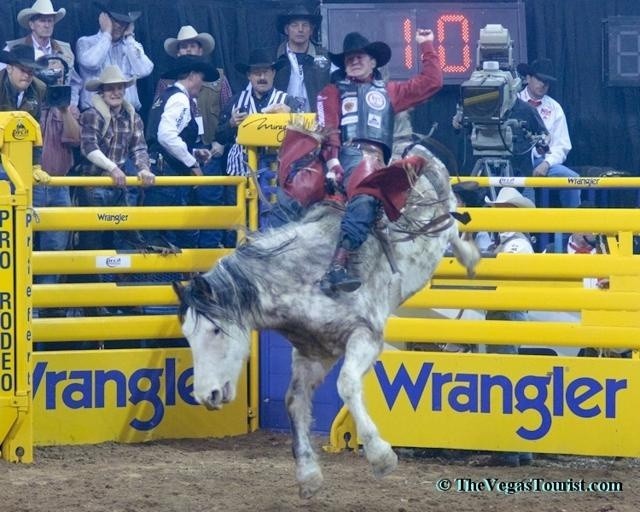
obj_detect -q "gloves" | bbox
[325,158,345,183]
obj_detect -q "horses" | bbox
[170,139,481,501]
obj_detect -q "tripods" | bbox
[469,119,553,244]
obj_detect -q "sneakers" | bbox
[321,268,362,295]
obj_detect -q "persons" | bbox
[0,1,639,358]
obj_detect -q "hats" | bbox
[485,186,535,207]
[86,64,135,91]
[451,181,489,209]
[159,53,220,81]
[518,57,559,82]
[327,31,391,70]
[98,1,149,23]
[235,47,289,76]
[0,45,44,69]
[163,25,215,58]
[277,5,323,35]
[17,1,66,29]
